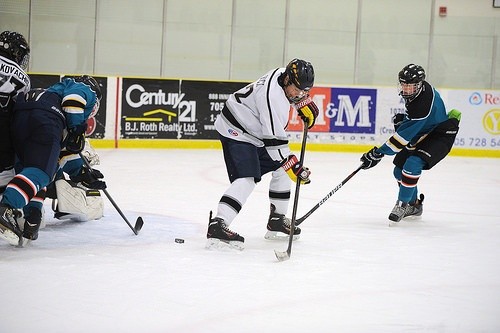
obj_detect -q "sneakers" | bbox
[206,210,245,252]
[388,201,407,227]
[265,204,301,240]
[0,203,23,246]
[19,209,43,247]
[403,194,424,218]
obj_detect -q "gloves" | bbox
[280,155,311,185]
[74,167,106,189]
[393,113,406,132]
[294,97,319,128]
[359,146,384,169]
[64,121,88,153]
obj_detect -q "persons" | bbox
[0,30,104,223]
[206,57,320,253]
[0,74,106,247]
[361,63,461,227]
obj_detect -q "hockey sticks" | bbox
[295,164,365,226]
[274,122,308,261]
[78,151,144,235]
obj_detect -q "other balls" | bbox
[175,239,184,243]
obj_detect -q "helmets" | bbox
[0,31,30,70]
[398,64,425,100]
[286,58,315,103]
[76,74,101,99]
